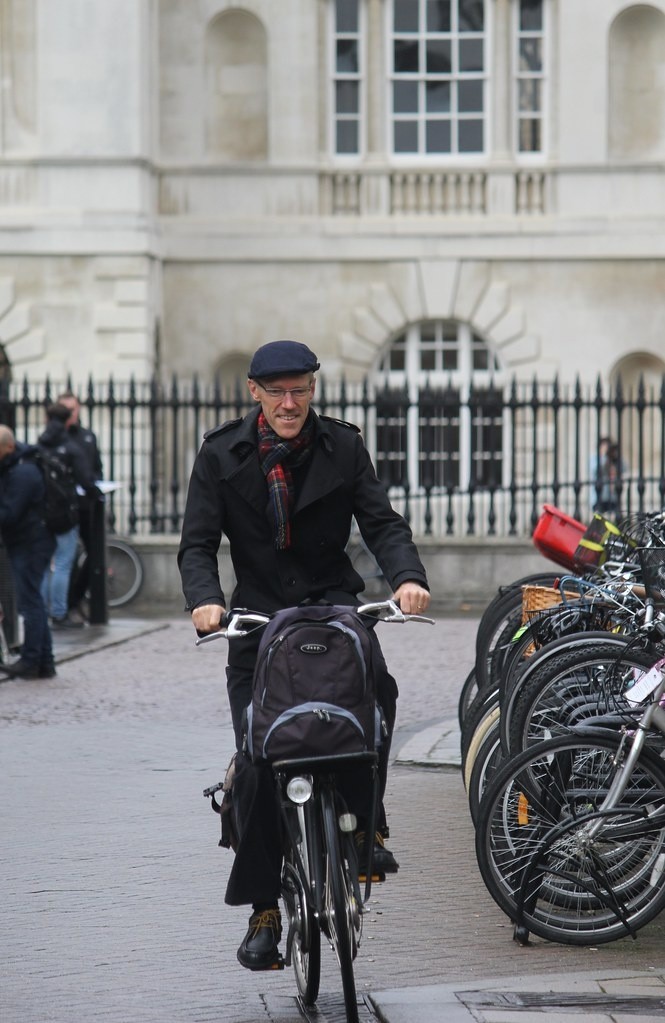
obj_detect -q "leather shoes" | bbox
[236,908,283,971]
[345,829,399,874]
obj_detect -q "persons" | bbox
[35,403,84,632]
[58,393,109,630]
[0,425,81,679]
[176,341,432,971]
[591,438,623,523]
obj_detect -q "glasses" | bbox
[255,380,312,395]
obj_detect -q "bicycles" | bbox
[78,539,142,609]
[194,597,437,1023]
[346,526,395,605]
[458,502,664,947]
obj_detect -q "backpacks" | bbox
[6,446,79,535]
[248,592,389,770]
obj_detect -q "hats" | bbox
[247,339,320,378]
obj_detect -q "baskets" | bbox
[635,545,665,602]
[603,530,647,572]
[525,601,615,652]
[569,512,639,570]
[518,583,611,662]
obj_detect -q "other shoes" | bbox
[1,653,56,680]
[49,614,86,632]
